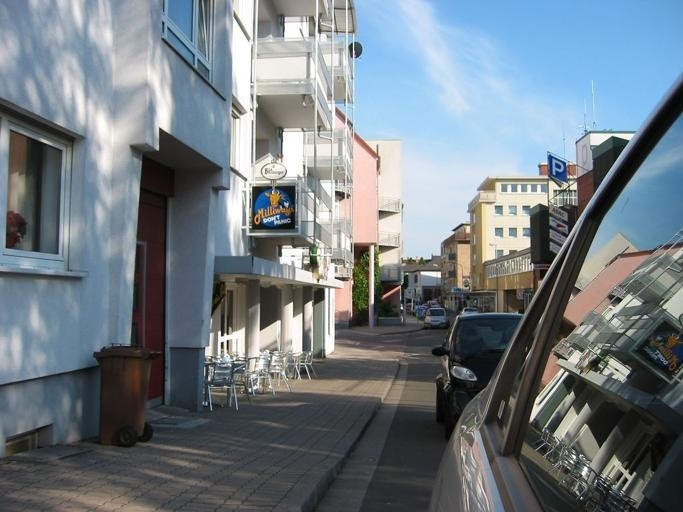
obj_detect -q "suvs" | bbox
[430,73,683,512]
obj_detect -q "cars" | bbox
[416,300,450,329]
[432,313,522,424]
[461,307,479,314]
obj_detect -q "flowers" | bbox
[5,209,29,250]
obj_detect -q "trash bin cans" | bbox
[93,343,160,447]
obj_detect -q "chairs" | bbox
[202,349,320,413]
[531,428,638,512]
